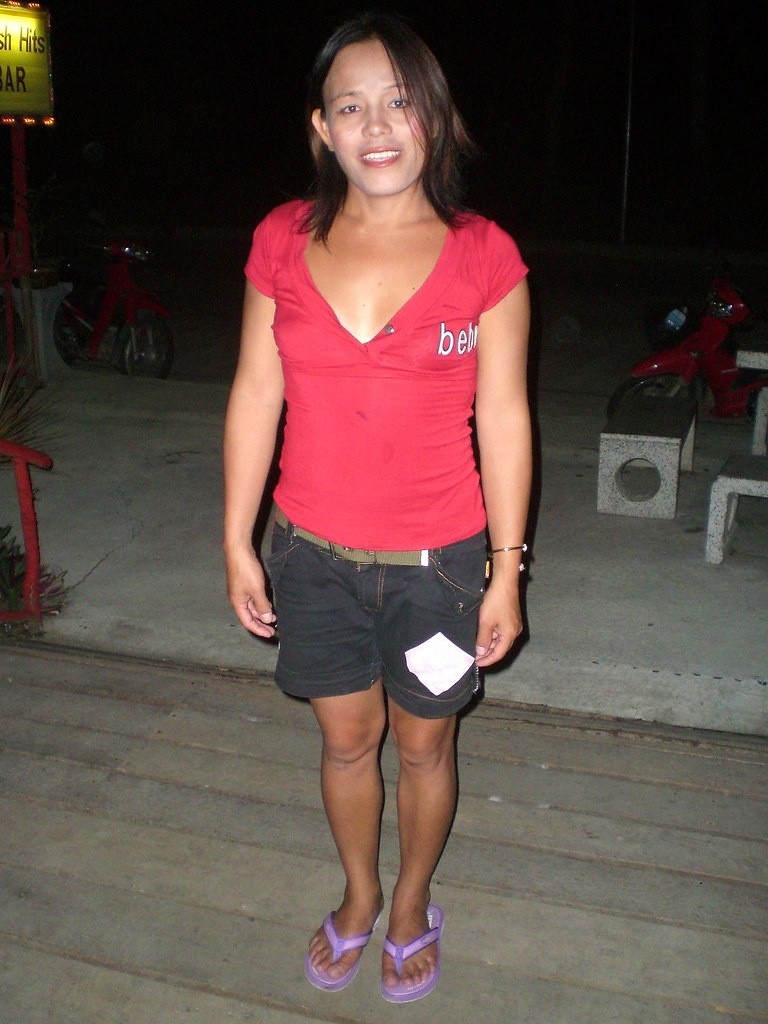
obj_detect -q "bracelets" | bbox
[487,543,527,571]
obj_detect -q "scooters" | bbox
[608,250,768,423]
[32,231,175,381]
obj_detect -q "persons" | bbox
[218,16,533,1005]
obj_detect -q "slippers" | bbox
[301,903,382,994]
[378,904,446,1004]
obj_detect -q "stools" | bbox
[595,394,699,521]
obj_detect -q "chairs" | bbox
[704,386,768,565]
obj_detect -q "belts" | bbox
[273,507,431,570]
